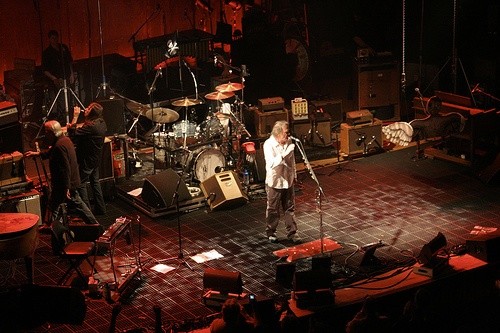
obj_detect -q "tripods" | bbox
[154,164,198,271]
[36,29,88,140]
[96,8,116,99]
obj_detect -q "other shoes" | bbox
[268,234,278,241]
[93,210,106,215]
[286,235,302,243]
[39,225,51,231]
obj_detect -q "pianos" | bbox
[91,217,140,285]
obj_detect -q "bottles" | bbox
[244,169,249,185]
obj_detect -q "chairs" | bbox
[50,219,98,287]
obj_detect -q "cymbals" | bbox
[215,83,245,92]
[126,102,150,116]
[146,108,179,123]
[204,92,235,100]
[171,99,201,107]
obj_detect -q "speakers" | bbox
[98,140,114,181]
[0,100,42,226]
[466,230,500,262]
[203,269,242,293]
[95,96,126,136]
[250,59,401,183]
[141,167,192,208]
[35,285,86,325]
[198,170,249,210]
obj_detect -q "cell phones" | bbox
[249,294,255,304]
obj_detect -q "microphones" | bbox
[315,131,323,139]
[415,88,424,98]
[214,55,217,67]
[290,135,300,142]
[304,131,311,138]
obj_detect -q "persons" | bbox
[210,258,500,333]
[66,103,106,215]
[263,120,302,243]
[23,120,99,234]
[41,32,74,113]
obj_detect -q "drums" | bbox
[152,131,175,171]
[0,213,40,260]
[198,116,225,140]
[189,148,226,184]
[172,120,198,144]
